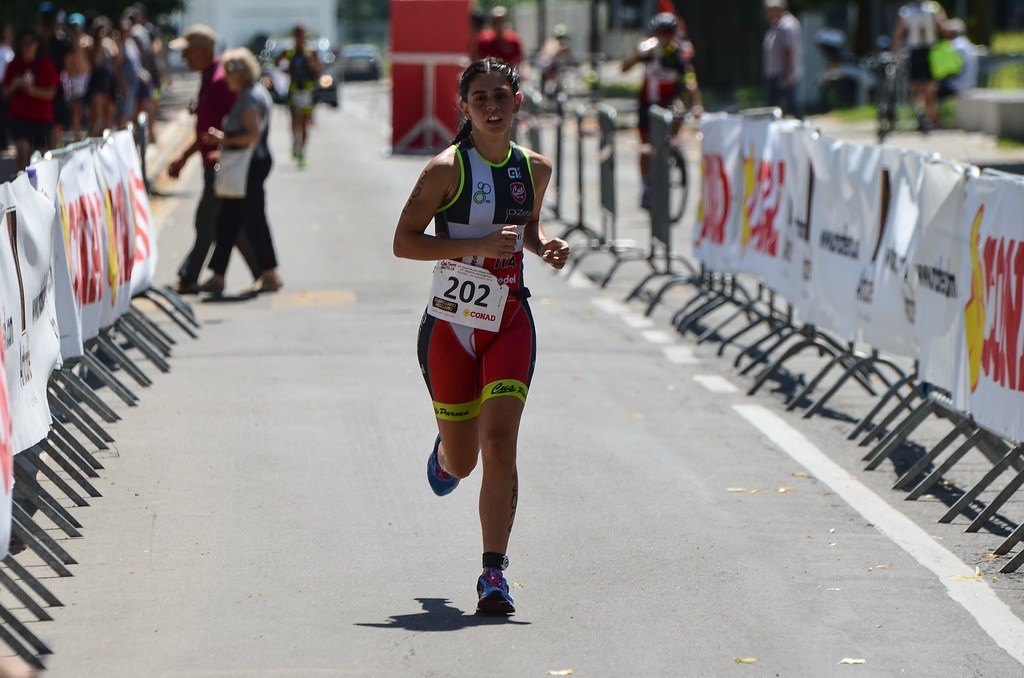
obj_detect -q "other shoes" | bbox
[165,280,200,295]
[198,277,223,293]
[240,273,281,297]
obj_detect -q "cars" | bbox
[258,36,339,107]
[341,45,383,80]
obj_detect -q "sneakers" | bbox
[427,432,461,497]
[476,567,515,612]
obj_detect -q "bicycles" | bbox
[645,107,698,226]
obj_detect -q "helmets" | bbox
[647,12,680,36]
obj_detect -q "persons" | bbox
[169,26,283,295]
[394,58,570,615]
[0,10,168,174]
[275,26,323,169]
[470,6,570,107]
[766,0,978,133]
[621,15,704,204]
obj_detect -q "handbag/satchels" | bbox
[213,147,253,199]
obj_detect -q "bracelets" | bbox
[537,238,548,255]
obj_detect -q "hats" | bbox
[169,25,215,54]
